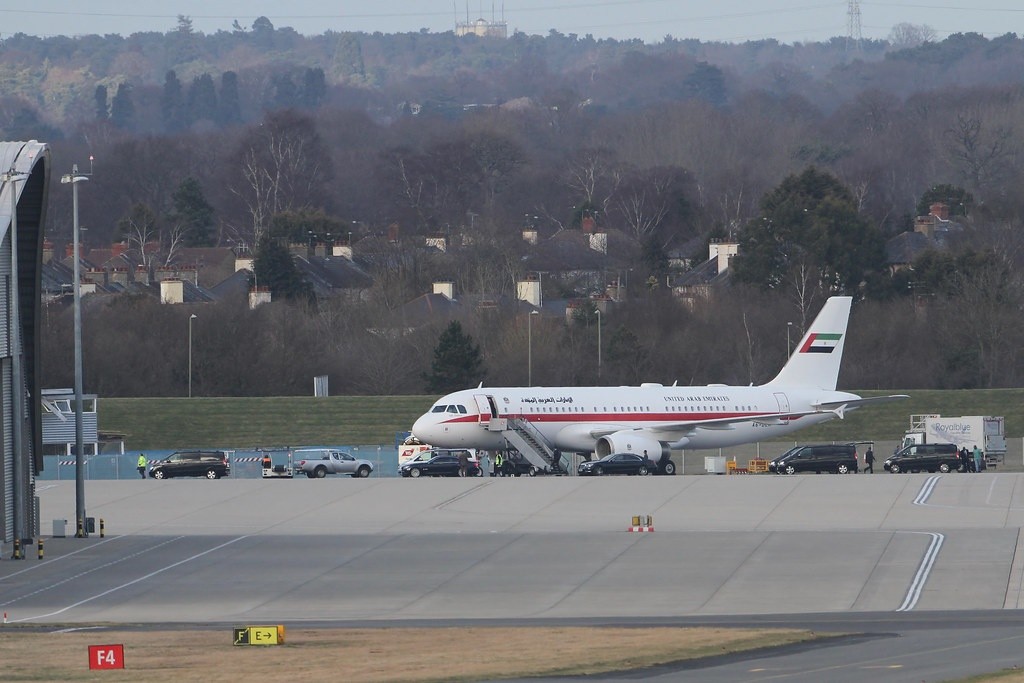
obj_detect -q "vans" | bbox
[149,450,230,480]
[768,444,857,475]
[398,447,478,476]
[884,444,962,473]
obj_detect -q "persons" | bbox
[897,446,900,452]
[643,450,648,459]
[553,447,561,468]
[458,451,468,477]
[961,447,971,473]
[261,455,271,469]
[864,447,876,474]
[973,445,982,473]
[136,453,147,479]
[494,451,503,477]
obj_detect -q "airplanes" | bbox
[412,296,911,476]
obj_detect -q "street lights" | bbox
[528,310,538,387]
[188,313,197,397]
[594,310,601,386]
[788,322,792,360]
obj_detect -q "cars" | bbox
[578,453,658,476]
[500,458,543,477]
[402,456,479,478]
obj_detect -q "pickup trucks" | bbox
[293,452,374,478]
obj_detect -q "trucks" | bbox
[901,414,1007,471]
